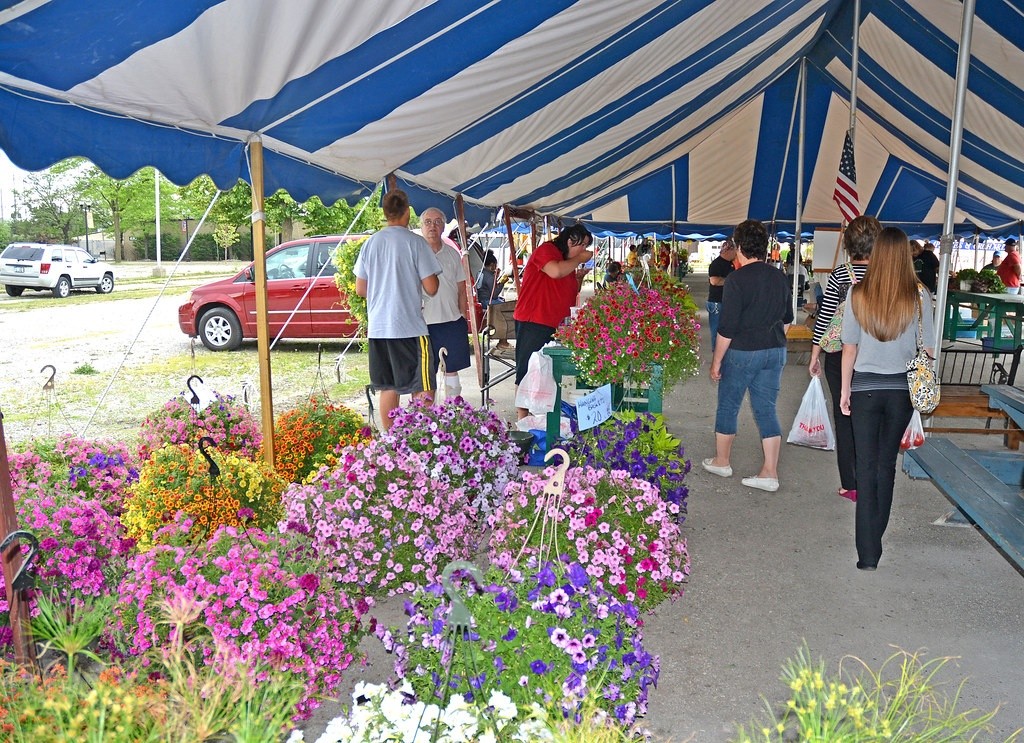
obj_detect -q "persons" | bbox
[809,215,884,502]
[839,227,936,571]
[512,225,593,421]
[984,251,1003,270]
[605,262,621,282]
[706,241,736,351]
[352,189,444,434]
[910,240,939,295]
[659,242,671,269]
[638,238,648,256]
[477,254,514,350]
[421,207,471,400]
[784,242,809,282]
[997,238,1023,294]
[628,244,639,265]
[702,220,794,492]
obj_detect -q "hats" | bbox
[1003,238,1016,246]
[994,251,1000,256]
[788,242,795,247]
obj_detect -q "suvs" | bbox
[1,242,113,298]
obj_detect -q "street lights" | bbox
[182,208,191,261]
[79,199,91,252]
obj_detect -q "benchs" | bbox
[904,437,1024,577]
[920,344,1024,451]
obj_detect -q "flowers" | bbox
[0,268,703,743]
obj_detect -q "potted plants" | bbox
[949,269,1008,294]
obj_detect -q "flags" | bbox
[831,131,860,222]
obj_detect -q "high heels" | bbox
[838,487,857,501]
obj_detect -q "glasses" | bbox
[424,219,441,226]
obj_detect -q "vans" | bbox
[470,232,524,281]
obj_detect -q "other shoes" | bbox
[497,343,513,349]
[742,474,779,492]
[857,561,877,571]
[702,457,732,477]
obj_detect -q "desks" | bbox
[543,345,665,467]
[948,295,1024,357]
[981,385,1024,422]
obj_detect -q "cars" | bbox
[178,221,504,352]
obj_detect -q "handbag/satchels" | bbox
[906,348,940,413]
[805,303,819,331]
[899,409,925,450]
[787,375,835,451]
[819,262,859,353]
